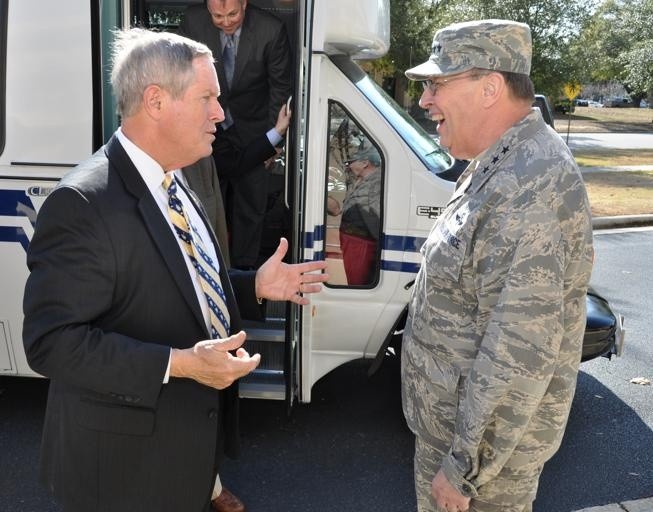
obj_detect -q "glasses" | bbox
[421,75,487,97]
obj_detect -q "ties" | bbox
[219,34,235,131]
[163,175,230,339]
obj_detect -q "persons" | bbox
[177,0,296,269]
[23,27,331,512]
[399,19,596,512]
[174,154,247,512]
[339,143,382,286]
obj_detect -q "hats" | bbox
[404,19,532,81]
[345,153,376,163]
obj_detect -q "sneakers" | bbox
[211,488,245,511]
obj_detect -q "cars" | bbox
[532,94,554,128]
[574,96,649,108]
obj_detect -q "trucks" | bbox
[0,0,626,435]
